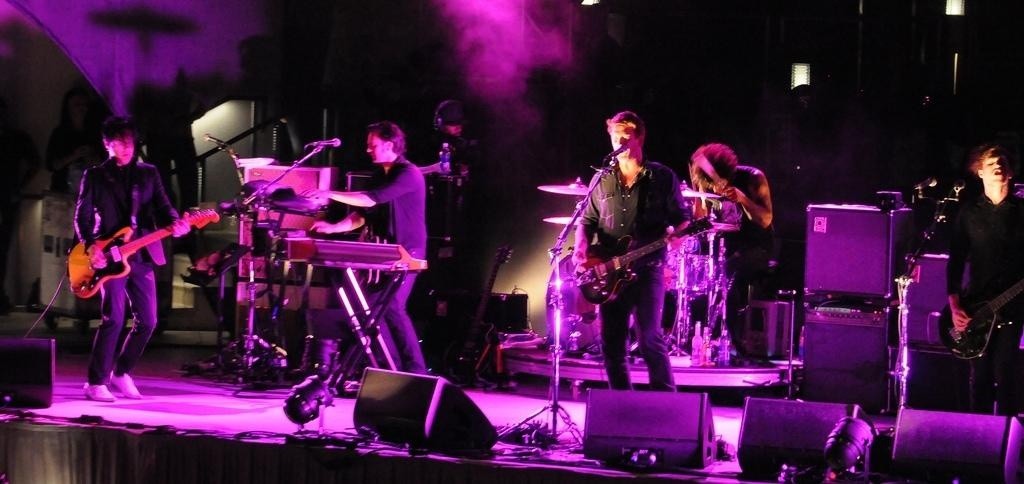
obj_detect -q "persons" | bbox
[72,116,191,402]
[573,110,710,391]
[691,141,779,364]
[944,142,1024,413]
[301,121,426,375]
[419,101,513,258]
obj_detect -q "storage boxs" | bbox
[346,171,385,235]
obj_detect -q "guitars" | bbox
[68,208,220,298]
[578,216,712,303]
[939,278,1024,359]
[442,242,514,375]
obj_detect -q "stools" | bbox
[745,300,794,359]
[743,260,778,341]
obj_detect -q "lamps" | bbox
[945,0,965,16]
[791,63,810,90]
[283,375,338,435]
[790,417,874,484]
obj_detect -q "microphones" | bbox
[312,137,342,148]
[912,176,938,190]
[607,144,627,159]
[203,133,230,148]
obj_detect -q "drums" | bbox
[667,250,712,296]
[547,253,641,354]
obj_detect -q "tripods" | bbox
[667,247,691,359]
[186,214,299,385]
[498,166,612,449]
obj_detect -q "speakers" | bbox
[353,367,499,452]
[582,389,720,469]
[803,304,890,414]
[892,408,1024,484]
[737,396,878,472]
[0,338,56,409]
[805,203,912,297]
[900,254,996,414]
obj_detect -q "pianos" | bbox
[281,237,429,272]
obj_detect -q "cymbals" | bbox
[536,182,592,195]
[542,216,579,225]
[681,190,724,199]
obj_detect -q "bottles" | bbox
[691,320,729,368]
[798,326,803,362]
[438,143,451,176]
[566,323,579,354]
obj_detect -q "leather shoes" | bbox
[110,370,143,398]
[83,383,117,401]
